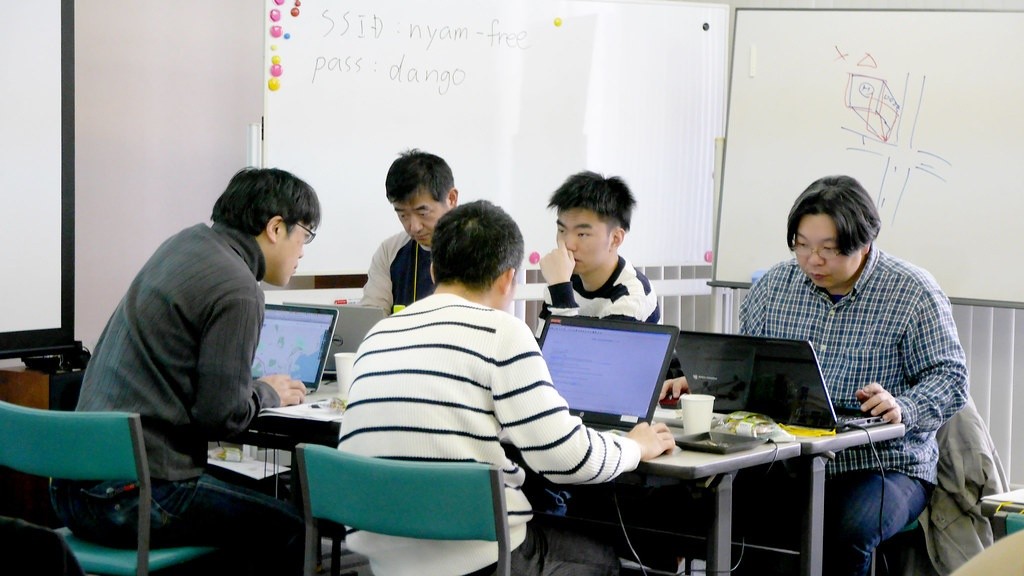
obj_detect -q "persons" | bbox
[356,152,459,319]
[534,170,682,380]
[659,175,970,576]
[54,170,320,576]
[338,201,675,576]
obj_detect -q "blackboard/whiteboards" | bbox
[706,6,1024,310]
[261,0,730,278]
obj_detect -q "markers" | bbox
[335,300,360,304]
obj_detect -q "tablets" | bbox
[673,431,768,454]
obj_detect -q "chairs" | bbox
[0,398,217,576]
[293,440,514,576]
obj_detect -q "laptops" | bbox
[252,304,339,395]
[538,315,680,433]
[282,301,383,381]
[675,331,891,433]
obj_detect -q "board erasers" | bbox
[753,268,769,282]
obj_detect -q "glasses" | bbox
[791,245,840,260]
[283,217,316,244]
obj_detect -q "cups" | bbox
[680,393,715,437]
[333,352,357,394]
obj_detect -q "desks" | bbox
[653,406,908,576]
[249,374,803,576]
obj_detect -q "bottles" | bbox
[393,304,405,314]
